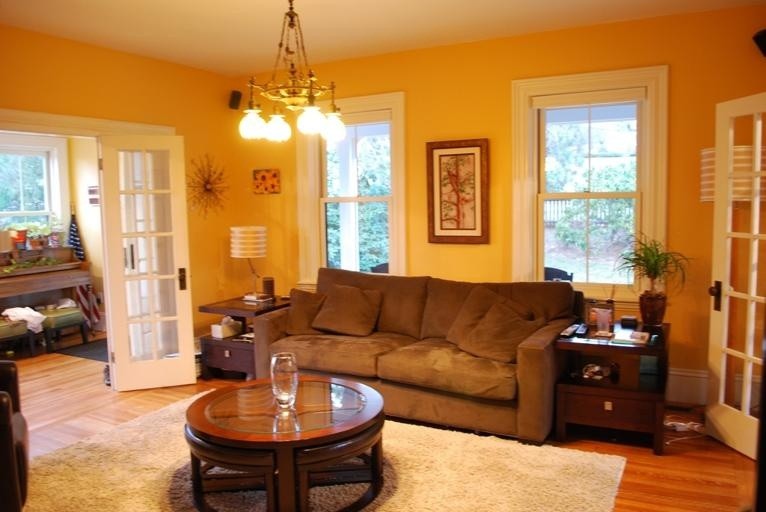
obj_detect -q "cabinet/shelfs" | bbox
[555,317,672,456]
[197,293,293,381]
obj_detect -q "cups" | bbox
[270,352,299,408]
[262,277,274,299]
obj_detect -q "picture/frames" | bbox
[425,139,490,243]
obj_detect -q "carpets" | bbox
[20,390,628,512]
[55,337,109,363]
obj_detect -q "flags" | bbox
[68,213,102,334]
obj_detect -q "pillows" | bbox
[445,283,547,364]
[285,284,383,338]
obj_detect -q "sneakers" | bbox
[103,365,109,386]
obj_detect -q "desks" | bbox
[0,261,94,338]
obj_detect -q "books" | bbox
[614,329,650,346]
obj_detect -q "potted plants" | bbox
[610,229,693,326]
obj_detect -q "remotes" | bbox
[559,324,580,337]
[576,323,589,335]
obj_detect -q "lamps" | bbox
[229,225,277,303]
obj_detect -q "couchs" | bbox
[253,268,585,448]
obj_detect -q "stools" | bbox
[0,307,89,358]
